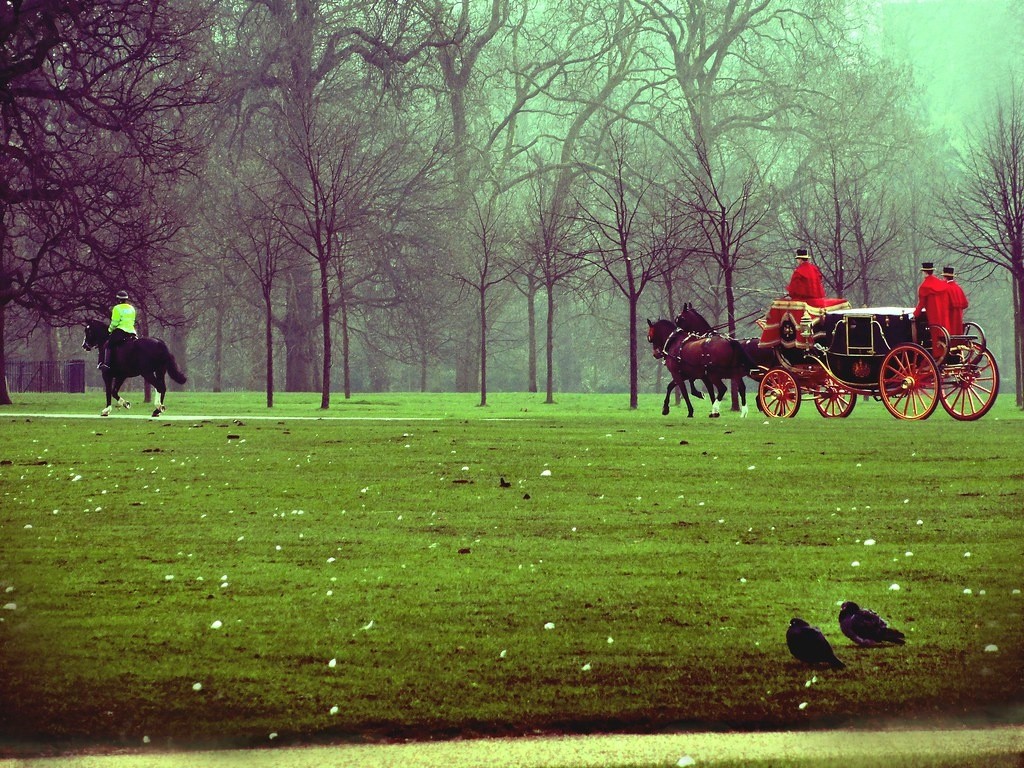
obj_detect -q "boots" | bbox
[99,348,110,369]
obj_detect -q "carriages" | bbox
[646,299,1000,421]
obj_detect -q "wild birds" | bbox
[786,618,847,668]
[838,602,906,646]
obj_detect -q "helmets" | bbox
[116,290,128,299]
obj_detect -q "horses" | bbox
[675,302,778,412]
[81,315,186,417]
[647,316,757,418]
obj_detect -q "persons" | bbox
[941,267,968,335]
[789,249,825,298]
[908,263,950,362]
[100,291,136,368]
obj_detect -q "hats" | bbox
[795,249,810,259]
[920,262,936,271]
[941,268,956,276]
